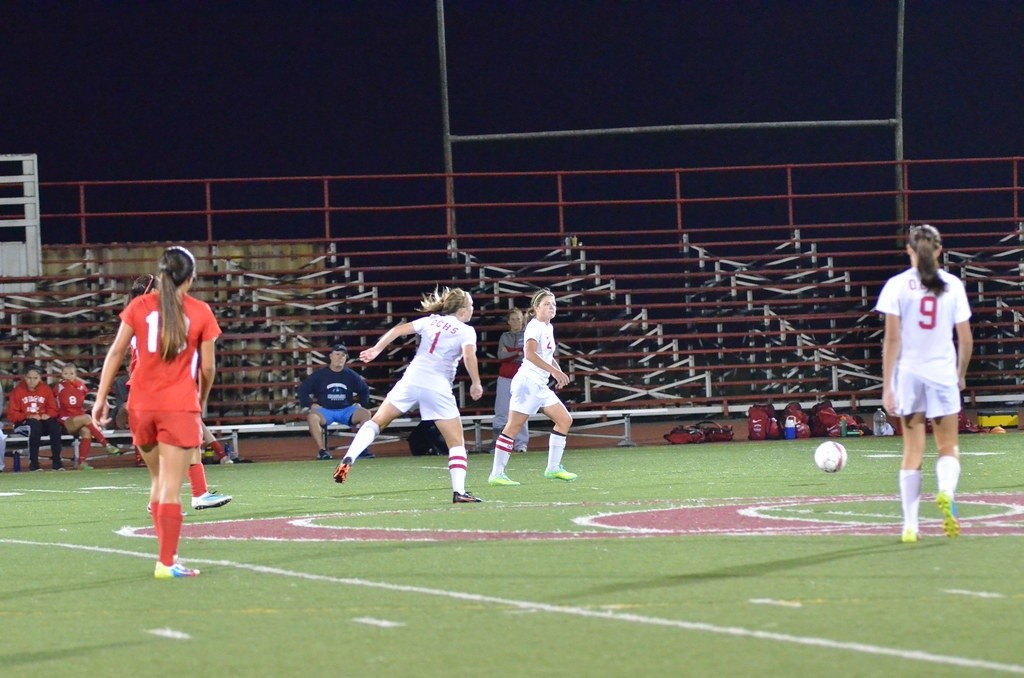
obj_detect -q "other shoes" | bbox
[317,448,332,459]
[51,460,66,471]
[359,448,375,458]
[480,439,496,454]
[136,460,147,467]
[219,455,233,464]
[28,462,44,473]
[77,461,94,471]
[103,443,120,454]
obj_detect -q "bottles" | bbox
[839,415,847,437]
[873,408,886,436]
[225,442,230,459]
[770,417,779,430]
[205,446,213,464]
[13,451,20,472]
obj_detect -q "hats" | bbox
[333,344,348,354]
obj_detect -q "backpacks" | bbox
[663,401,841,446]
[926,405,979,433]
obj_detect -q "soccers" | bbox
[815,441,847,473]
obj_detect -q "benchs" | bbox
[0,224,1024,472]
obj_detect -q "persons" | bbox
[0,363,120,472]
[298,345,375,458]
[488,289,578,486]
[488,307,529,453]
[875,224,974,542]
[92,246,233,578]
[333,288,483,502]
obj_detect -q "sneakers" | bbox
[902,529,922,543]
[935,493,960,539]
[147,503,187,516]
[453,492,483,503]
[191,490,232,511]
[543,465,578,481]
[155,562,200,579]
[172,555,182,565]
[332,456,352,483]
[489,474,521,487]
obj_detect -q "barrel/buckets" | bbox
[785,415,796,439]
